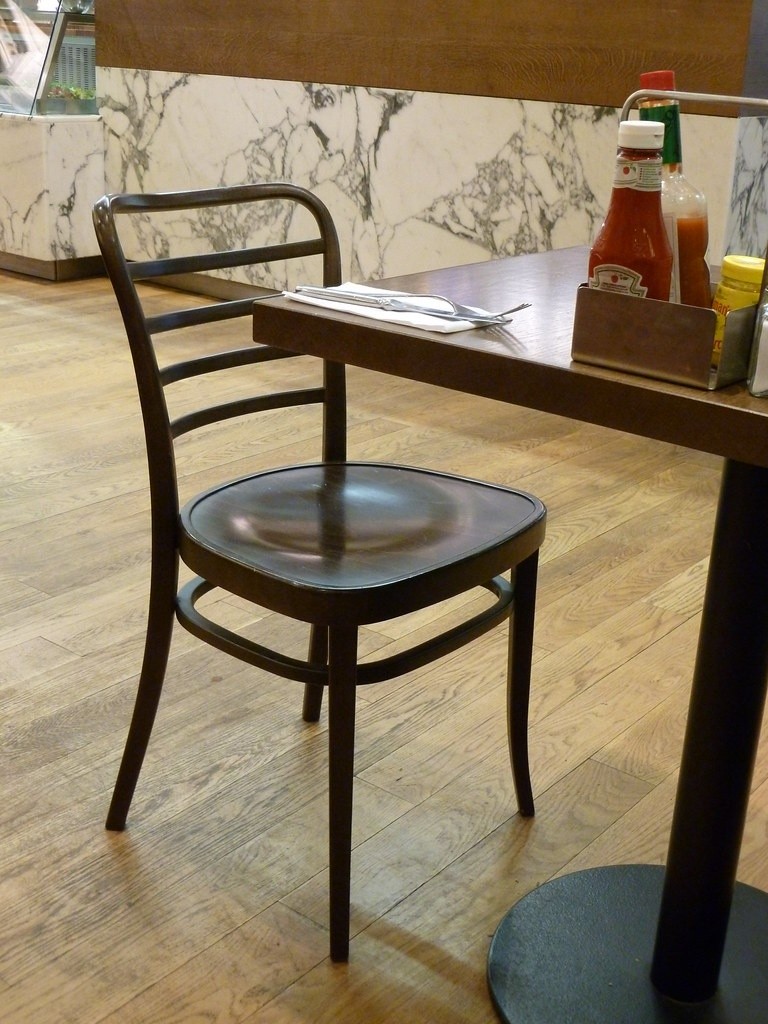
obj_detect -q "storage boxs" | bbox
[570,280,760,390]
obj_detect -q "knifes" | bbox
[295,286,514,325]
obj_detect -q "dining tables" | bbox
[253,242,768,1024]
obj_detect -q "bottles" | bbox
[710,255,766,369]
[586,120,674,302]
[638,70,712,308]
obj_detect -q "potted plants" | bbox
[35,82,97,116]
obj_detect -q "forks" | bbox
[302,285,533,318]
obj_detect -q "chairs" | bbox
[94,181,547,965]
